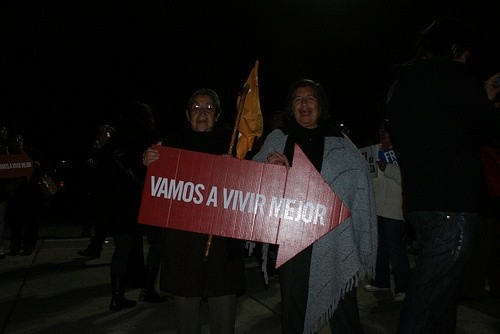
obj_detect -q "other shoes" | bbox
[78,248,100,258]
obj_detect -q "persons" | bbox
[0,126,46,259]
[77,39,500,334]
[246,78,379,334]
[141,88,251,334]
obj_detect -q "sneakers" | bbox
[365,285,390,291]
[393,292,406,301]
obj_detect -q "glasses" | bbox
[191,102,216,112]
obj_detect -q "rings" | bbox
[144,157,147,161]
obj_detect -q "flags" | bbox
[235,60,264,159]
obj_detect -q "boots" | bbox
[109,272,137,311]
[141,265,167,303]
